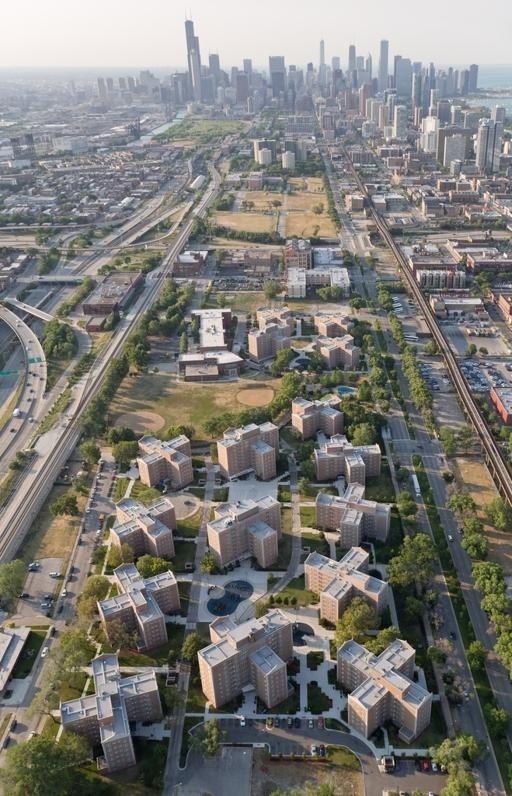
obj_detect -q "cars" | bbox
[392,296,441,390]
[448,527,463,542]
[311,744,325,756]
[414,759,446,773]
[9,319,119,659]
[459,362,512,393]
[464,691,469,702]
[240,716,324,730]
[449,630,457,641]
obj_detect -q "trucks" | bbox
[412,475,421,497]
